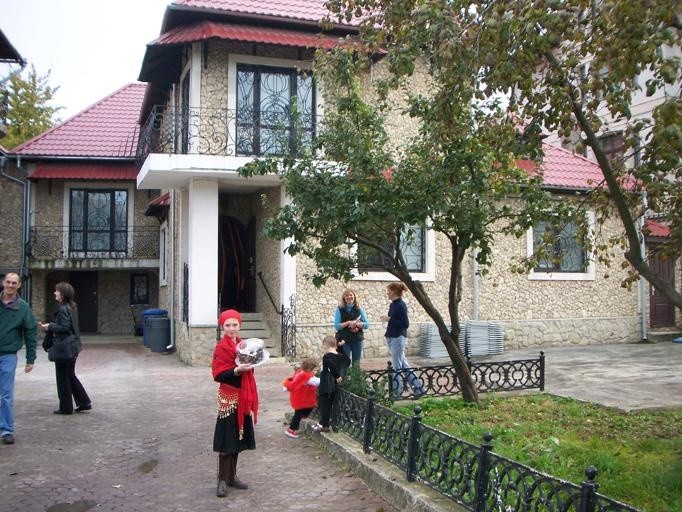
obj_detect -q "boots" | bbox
[216,455,232,497]
[229,453,248,489]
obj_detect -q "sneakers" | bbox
[53,410,72,414]
[75,404,91,411]
[311,422,328,432]
[285,428,299,438]
[412,392,425,400]
[3,434,14,443]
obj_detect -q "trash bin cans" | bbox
[143,313,167,347]
[147,317,170,352]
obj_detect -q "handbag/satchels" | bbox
[48,335,75,361]
[335,333,342,353]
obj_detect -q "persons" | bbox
[280,357,323,440]
[1,272,38,446]
[211,309,269,499]
[316,335,349,435]
[37,281,93,416]
[334,289,371,370]
[381,283,429,402]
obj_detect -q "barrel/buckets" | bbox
[147,318,170,353]
[142,309,168,346]
[145,315,167,349]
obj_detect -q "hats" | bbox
[220,310,241,328]
